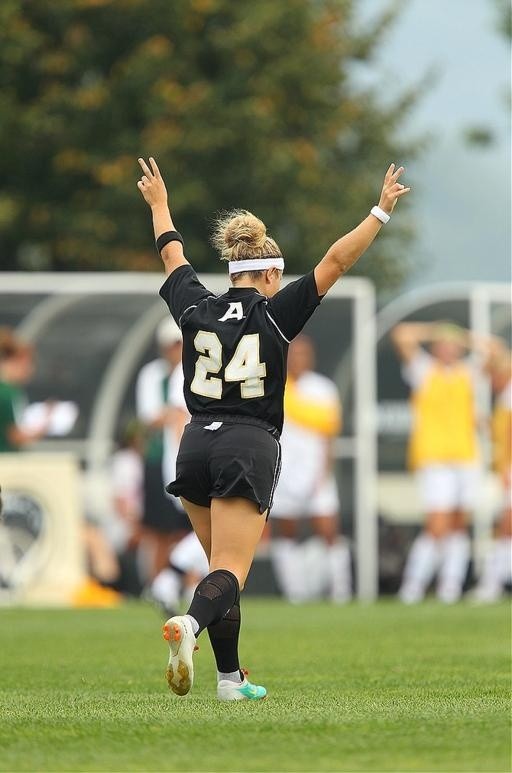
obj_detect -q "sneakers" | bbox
[138,585,199,697]
[216,670,266,702]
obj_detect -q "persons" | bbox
[0,313,512,616]
[136,157,413,703]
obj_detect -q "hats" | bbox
[156,316,182,351]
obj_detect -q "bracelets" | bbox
[370,206,390,224]
[156,231,184,256]
